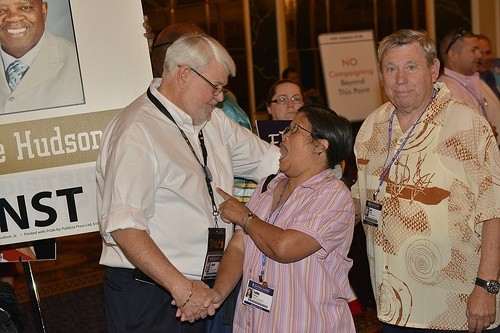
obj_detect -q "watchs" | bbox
[242,213,256,235]
[475,277,500,294]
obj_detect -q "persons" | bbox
[150,22,265,332]
[284,68,324,106]
[95,32,282,333]
[171,108,357,333]
[0,0,84,115]
[348,30,500,333]
[435,30,488,119]
[0,262,23,333]
[258,80,346,181]
[476,35,500,101]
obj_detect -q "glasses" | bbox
[271,95,304,103]
[283,123,315,136]
[177,64,229,96]
[445,27,470,54]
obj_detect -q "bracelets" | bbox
[179,282,195,309]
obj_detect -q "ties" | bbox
[6,60,29,91]
